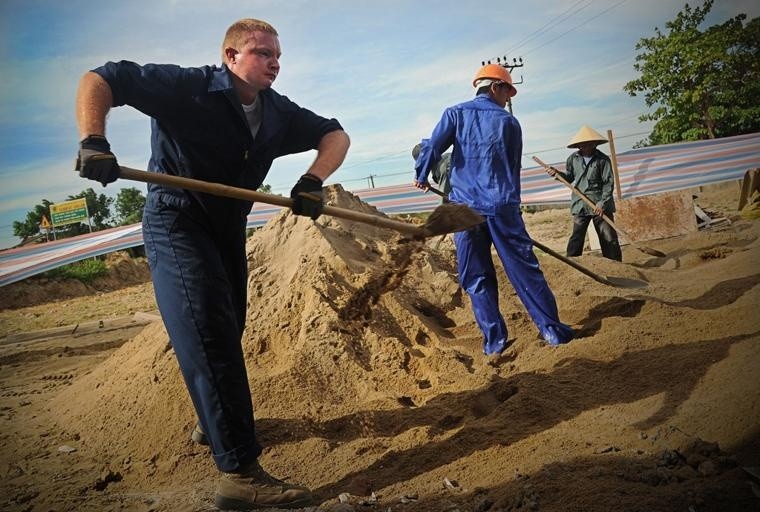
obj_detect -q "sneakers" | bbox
[190,420,265,457]
[212,459,315,511]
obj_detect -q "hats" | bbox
[566,124,609,149]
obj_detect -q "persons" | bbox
[412,141,472,247]
[72,17,351,507]
[548,125,624,264]
[417,67,584,355]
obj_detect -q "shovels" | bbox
[73,158,487,238]
[429,187,649,289]
[532,158,666,258]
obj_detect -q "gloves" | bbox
[289,172,326,221]
[77,133,122,190]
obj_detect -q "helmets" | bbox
[472,63,518,98]
